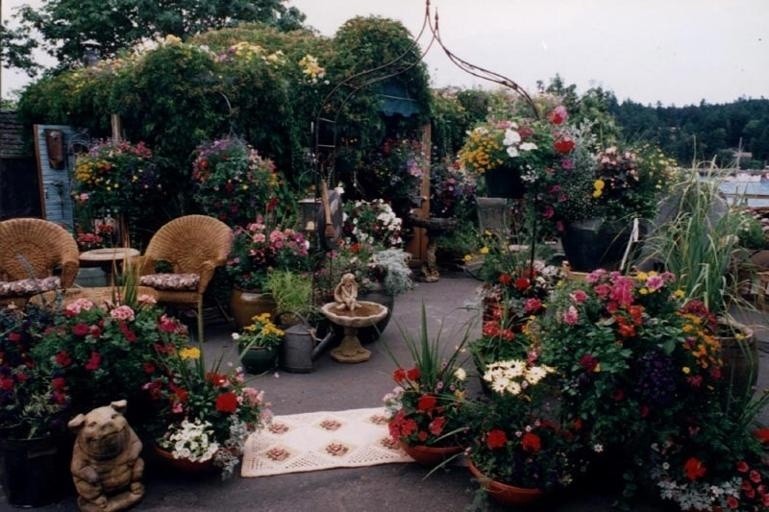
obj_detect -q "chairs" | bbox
[139,212,234,342]
[0,217,81,296]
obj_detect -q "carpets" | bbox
[239,406,417,479]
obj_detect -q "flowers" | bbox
[65,134,175,211]
[0,300,164,460]
[457,106,673,218]
[146,313,285,465]
[237,315,281,348]
[461,227,769,512]
[193,136,475,286]
[374,299,478,445]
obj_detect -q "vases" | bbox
[153,438,221,484]
[403,441,463,466]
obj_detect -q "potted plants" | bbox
[639,132,768,424]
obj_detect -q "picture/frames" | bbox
[239,339,274,376]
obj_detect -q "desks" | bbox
[79,246,140,288]
